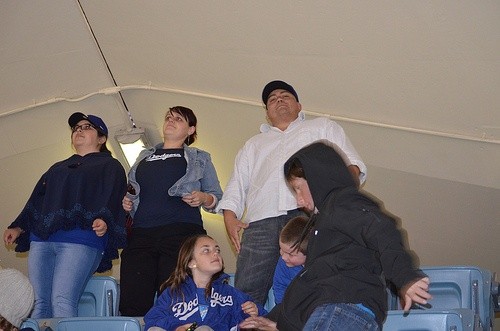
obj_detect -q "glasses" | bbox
[71,124,98,131]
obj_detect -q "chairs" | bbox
[20,266,493,331]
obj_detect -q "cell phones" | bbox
[186,322,198,331]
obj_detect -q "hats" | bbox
[67,111,108,137]
[261,80,298,105]
